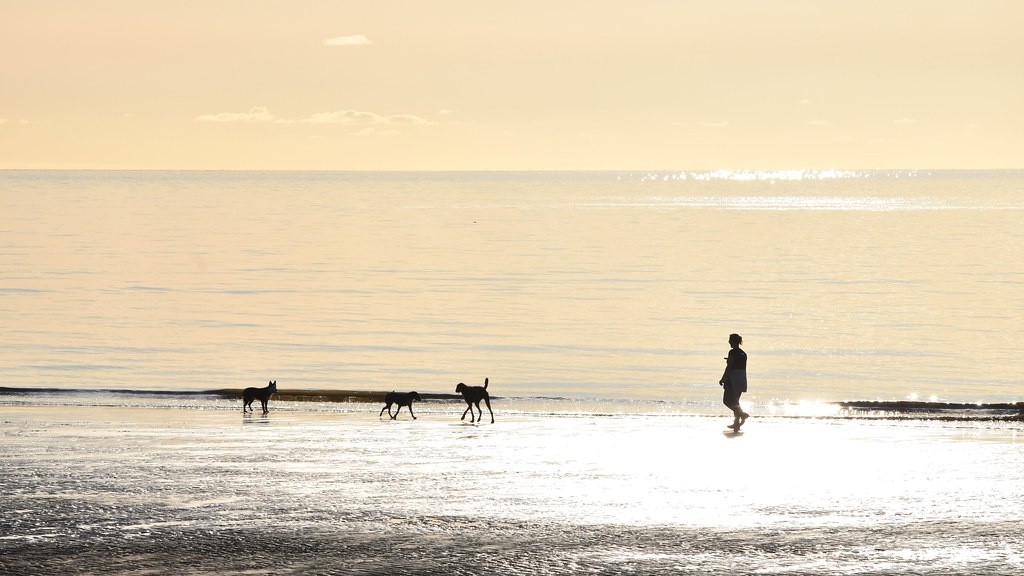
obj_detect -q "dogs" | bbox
[380,391,421,420]
[242,381,278,413]
[456,378,495,423]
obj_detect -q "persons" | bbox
[720,333,749,429]
